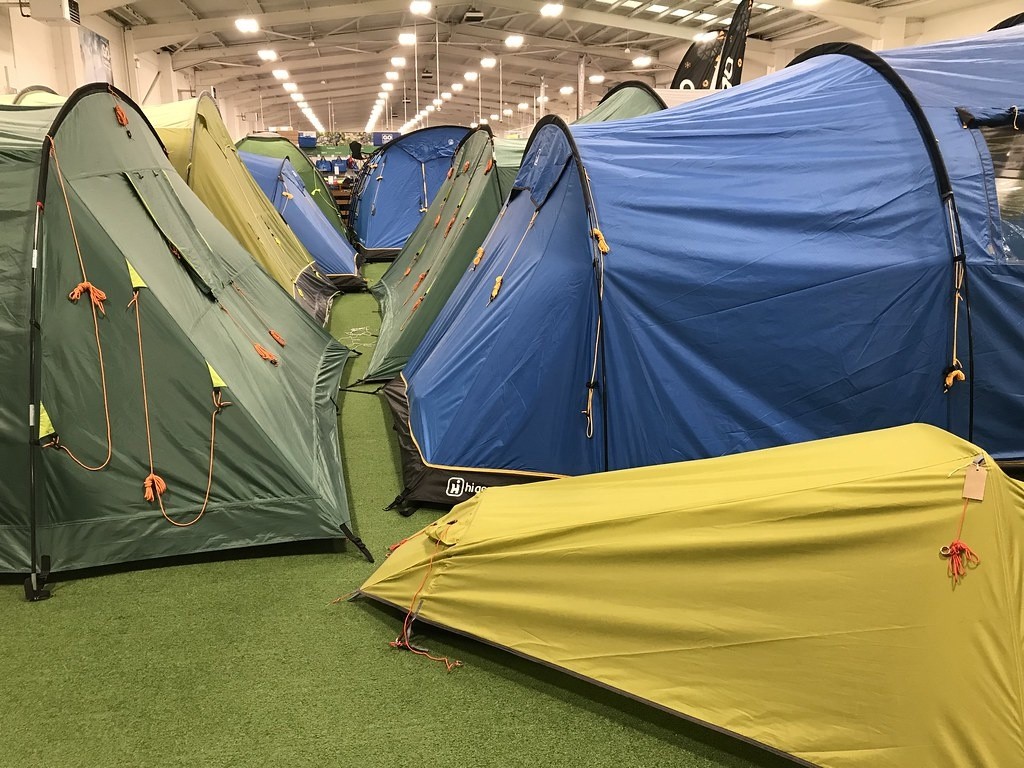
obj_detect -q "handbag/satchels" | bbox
[316,156,331,171]
[332,156,347,171]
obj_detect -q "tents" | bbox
[371,11,1024,520]
[344,123,474,260]
[234,131,350,246]
[1,82,347,330]
[339,80,726,394]
[1,81,376,603]
[329,422,1024,766]
[236,150,370,293]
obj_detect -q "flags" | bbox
[669,28,728,89]
[715,0,754,89]
